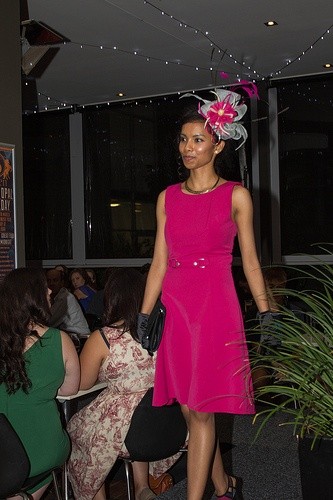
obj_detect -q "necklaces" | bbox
[185,176,220,194]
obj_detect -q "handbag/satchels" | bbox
[147,298,166,352]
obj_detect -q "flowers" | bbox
[178,72,259,151]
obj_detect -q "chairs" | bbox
[117,387,188,500]
[0,413,61,500]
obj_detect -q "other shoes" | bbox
[216,475,237,500]
[138,488,158,500]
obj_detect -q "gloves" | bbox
[254,311,279,355]
[130,311,152,349]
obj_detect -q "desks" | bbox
[55,383,108,500]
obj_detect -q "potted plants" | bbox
[225,242,333,500]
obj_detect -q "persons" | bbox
[137,73,279,500]
[0,265,304,500]
